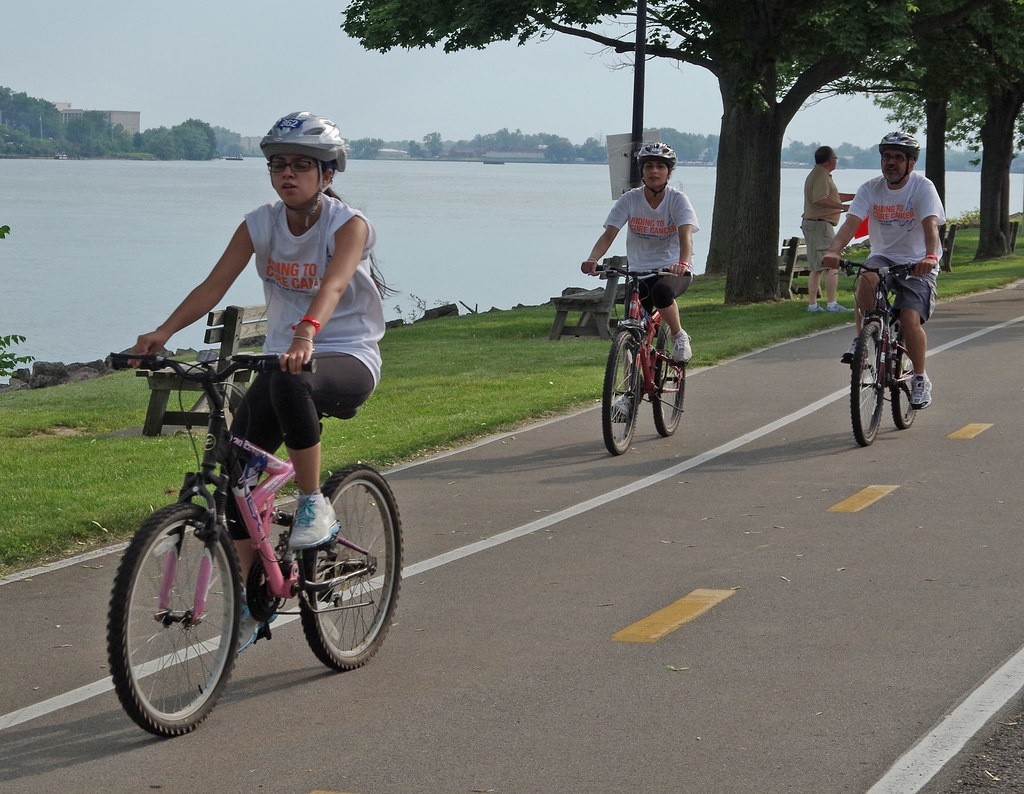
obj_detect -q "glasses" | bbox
[267,160,322,173]
[834,157,837,160]
[881,154,914,163]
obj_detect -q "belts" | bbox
[804,219,834,226]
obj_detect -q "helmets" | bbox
[879,132,920,162]
[260,112,346,172]
[637,142,677,169]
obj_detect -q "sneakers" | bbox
[827,303,854,313]
[235,599,278,654]
[842,338,869,360]
[612,386,633,419]
[808,303,827,313]
[910,376,932,409]
[288,486,340,549]
[671,327,692,365]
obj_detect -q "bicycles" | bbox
[584,263,692,456]
[816,256,929,447]
[103,353,406,737]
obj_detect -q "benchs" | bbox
[551,255,634,342]
[937,223,957,273]
[776,236,824,299]
[136,305,274,439]
[1009,222,1020,253]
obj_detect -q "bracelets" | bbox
[925,255,938,261]
[292,335,313,343]
[292,315,320,336]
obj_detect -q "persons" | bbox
[581,141,700,423]
[822,131,946,411]
[800,145,856,312]
[127,110,402,655]
